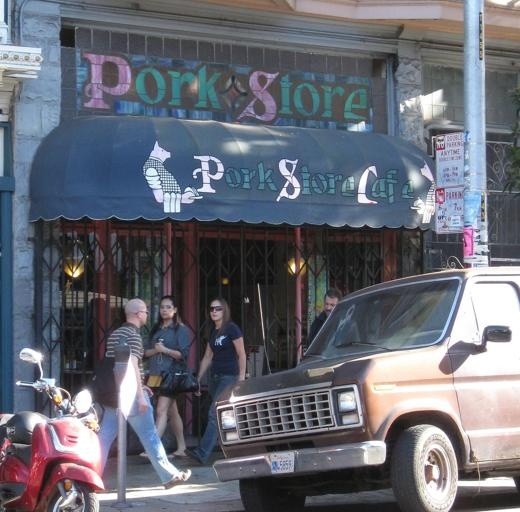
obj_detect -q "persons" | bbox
[96,297,193,491]
[137,294,191,462]
[180,296,248,466]
[307,287,341,349]
[84,297,108,369]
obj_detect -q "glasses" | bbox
[208,306,223,312]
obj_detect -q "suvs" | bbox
[206,261,519,512]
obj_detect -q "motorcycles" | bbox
[0,346,106,512]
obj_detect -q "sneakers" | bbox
[163,469,192,490]
[139,448,205,464]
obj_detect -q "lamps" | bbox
[63,246,86,279]
[288,244,307,277]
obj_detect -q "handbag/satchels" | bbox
[92,354,119,409]
[163,368,201,394]
[144,371,164,390]
